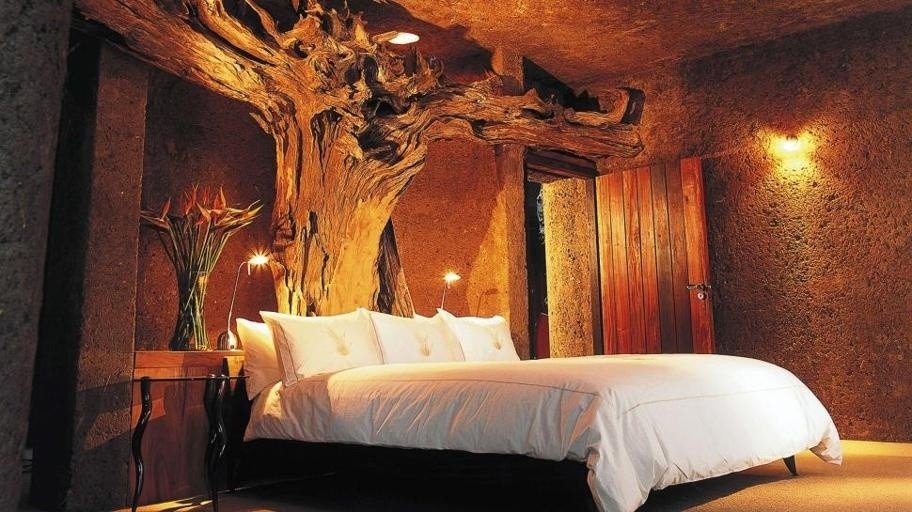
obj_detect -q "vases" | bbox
[168,270,213,351]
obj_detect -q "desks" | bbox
[130,374,249,511]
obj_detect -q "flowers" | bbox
[143,181,265,347]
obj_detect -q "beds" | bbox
[217,352,799,511]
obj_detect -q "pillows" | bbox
[233,305,522,400]
